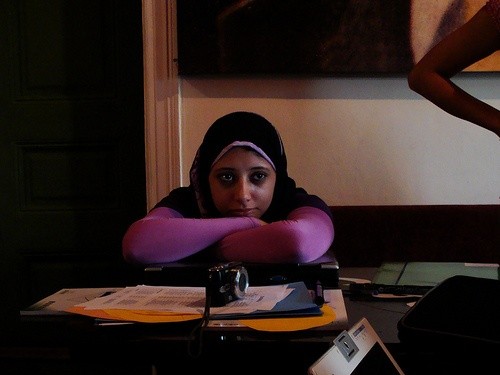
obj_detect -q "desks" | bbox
[0,267,421,375]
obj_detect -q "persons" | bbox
[122,111,333,270]
[408,0,500,139]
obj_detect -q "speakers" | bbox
[398,275,500,356]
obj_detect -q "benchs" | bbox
[327,205,500,267]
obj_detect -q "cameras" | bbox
[206,261,248,306]
